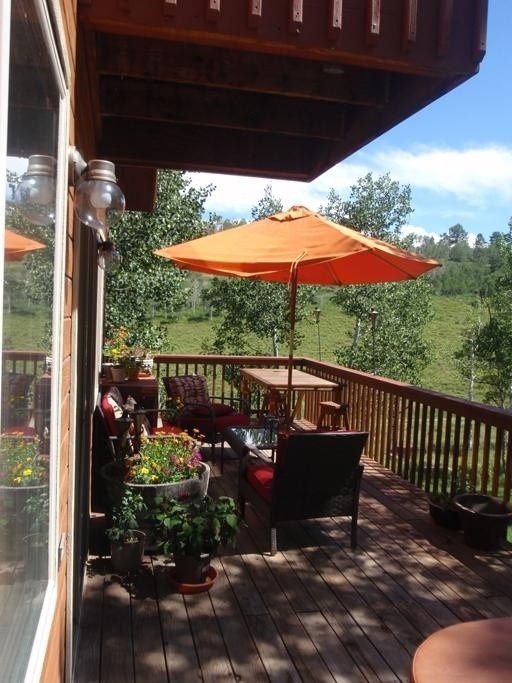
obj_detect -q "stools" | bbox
[317,402,349,431]
[261,393,287,418]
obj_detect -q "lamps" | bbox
[97,241,122,272]
[75,159,126,231]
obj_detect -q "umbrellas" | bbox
[150,203,442,434]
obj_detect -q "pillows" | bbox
[188,404,234,416]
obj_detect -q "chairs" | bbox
[240,433,369,555]
[93,387,184,461]
[161,376,252,465]
[1,373,32,427]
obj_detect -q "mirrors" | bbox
[0,0,63,682]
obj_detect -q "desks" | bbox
[239,368,348,431]
[412,617,512,683]
[34,375,51,428]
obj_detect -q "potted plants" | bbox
[101,326,153,380]
[20,494,48,579]
[151,489,246,582]
[105,490,148,571]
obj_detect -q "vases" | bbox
[105,463,211,511]
[3,485,46,514]
[427,466,476,529]
[454,494,512,551]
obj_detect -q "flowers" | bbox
[126,428,206,484]
[0,434,50,488]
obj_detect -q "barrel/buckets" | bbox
[99,458,210,553]
[453,491,512,549]
[113,528,144,569]
